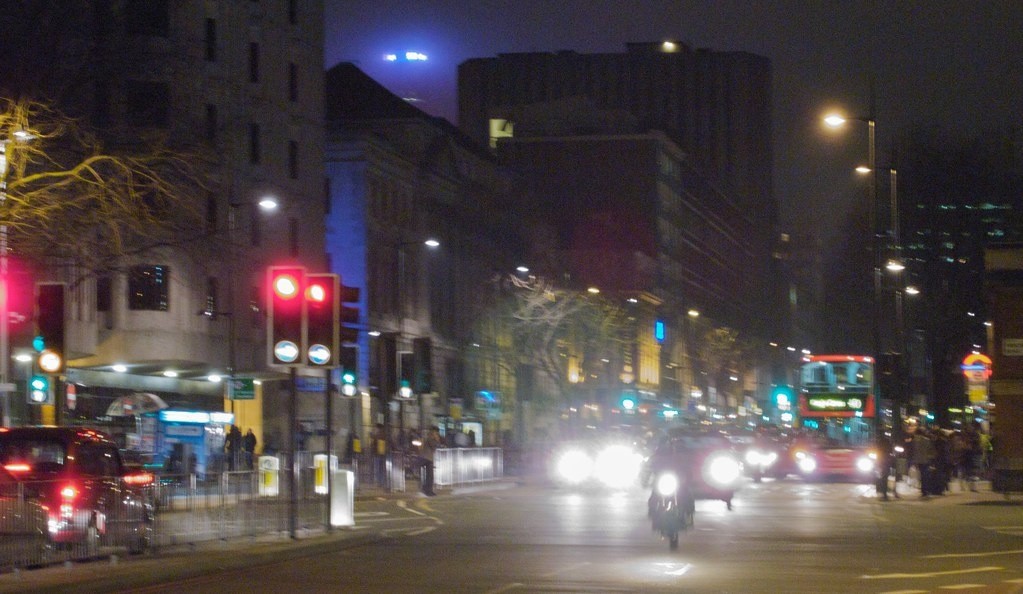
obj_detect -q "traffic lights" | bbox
[27,282,65,404]
[304,272,340,369]
[267,266,307,365]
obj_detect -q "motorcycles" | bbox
[648,468,694,548]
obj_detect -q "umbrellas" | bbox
[105,392,169,441]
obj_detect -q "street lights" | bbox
[822,112,920,450]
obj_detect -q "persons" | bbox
[224,423,308,476]
[640,420,982,517]
[369,423,475,498]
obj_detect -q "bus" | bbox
[794,355,880,482]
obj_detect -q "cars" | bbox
[0,426,155,566]
[524,423,796,522]
